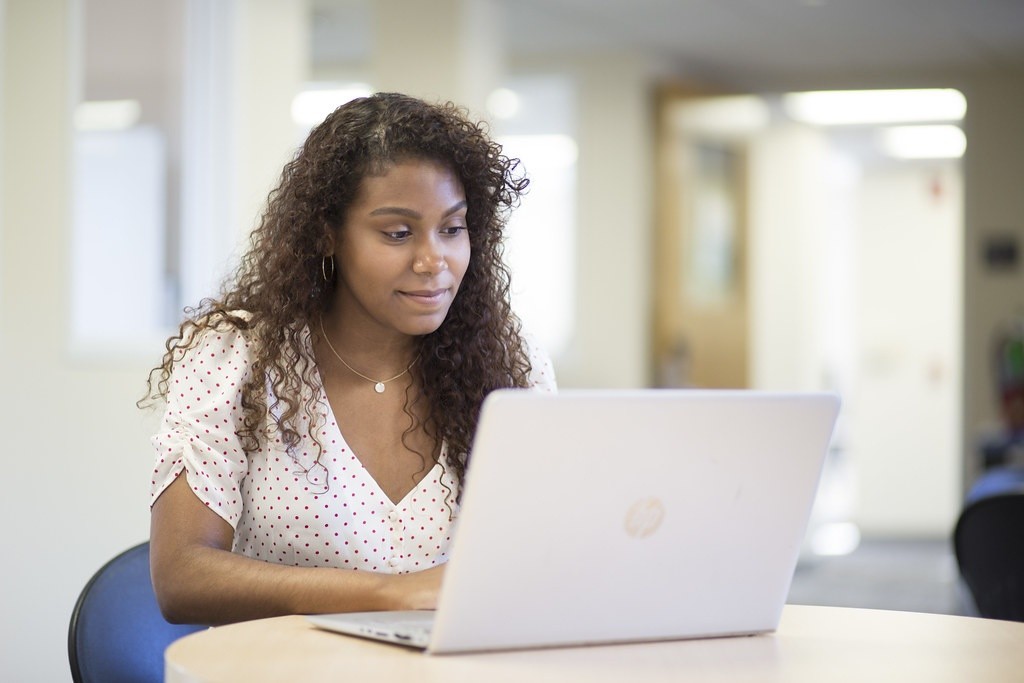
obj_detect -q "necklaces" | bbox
[320,313,425,393]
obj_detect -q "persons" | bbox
[137,92,558,628]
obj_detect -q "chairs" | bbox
[950,467,1024,622]
[67,541,208,683]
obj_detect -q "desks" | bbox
[164,604,1024,683]
